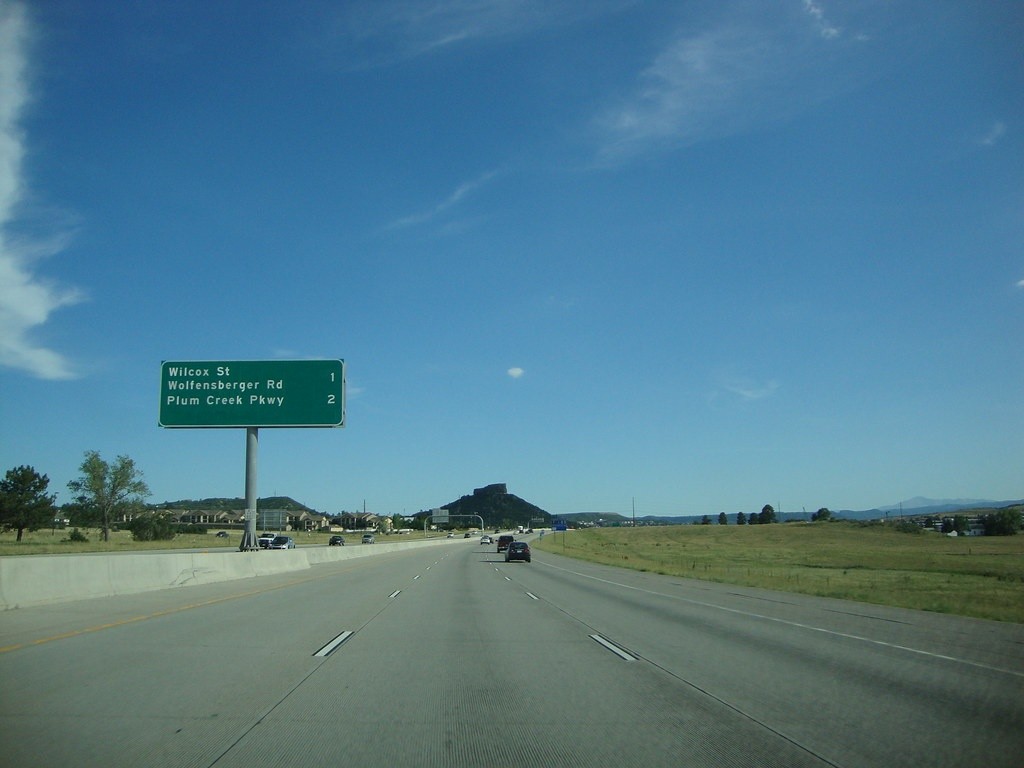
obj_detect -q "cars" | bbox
[504,542,531,562]
[328,536,346,546]
[513,526,534,535]
[215,531,229,537]
[472,530,477,535]
[494,529,500,534]
[480,535,494,545]
[268,536,295,550]
[464,533,471,538]
[361,534,375,544]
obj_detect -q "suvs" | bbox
[447,532,454,538]
[257,532,277,547]
[495,535,516,553]
